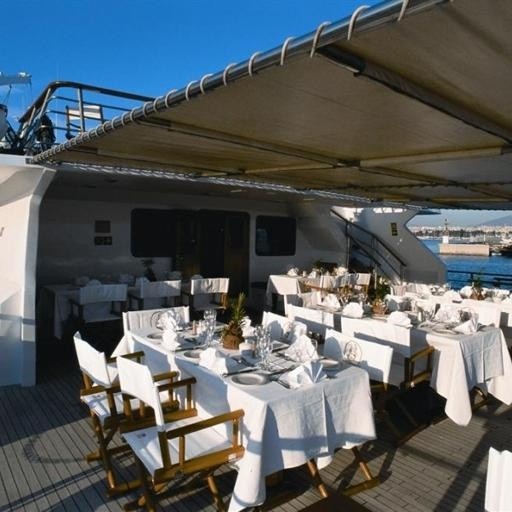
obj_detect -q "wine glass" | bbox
[253,324,272,368]
[196,307,217,348]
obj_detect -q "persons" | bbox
[0,104,56,155]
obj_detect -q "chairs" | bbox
[73,329,180,497]
[66,102,105,139]
[261,310,308,343]
[322,327,400,438]
[115,355,245,512]
[181,277,230,316]
[122,305,190,335]
[264,273,511,428]
[139,280,182,310]
[72,284,144,328]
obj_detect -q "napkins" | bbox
[285,335,327,362]
[156,309,183,331]
[199,346,239,376]
[289,361,328,390]
[238,315,256,336]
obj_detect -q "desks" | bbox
[109,322,378,512]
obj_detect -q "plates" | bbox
[432,328,459,337]
[147,328,267,391]
[317,357,340,376]
